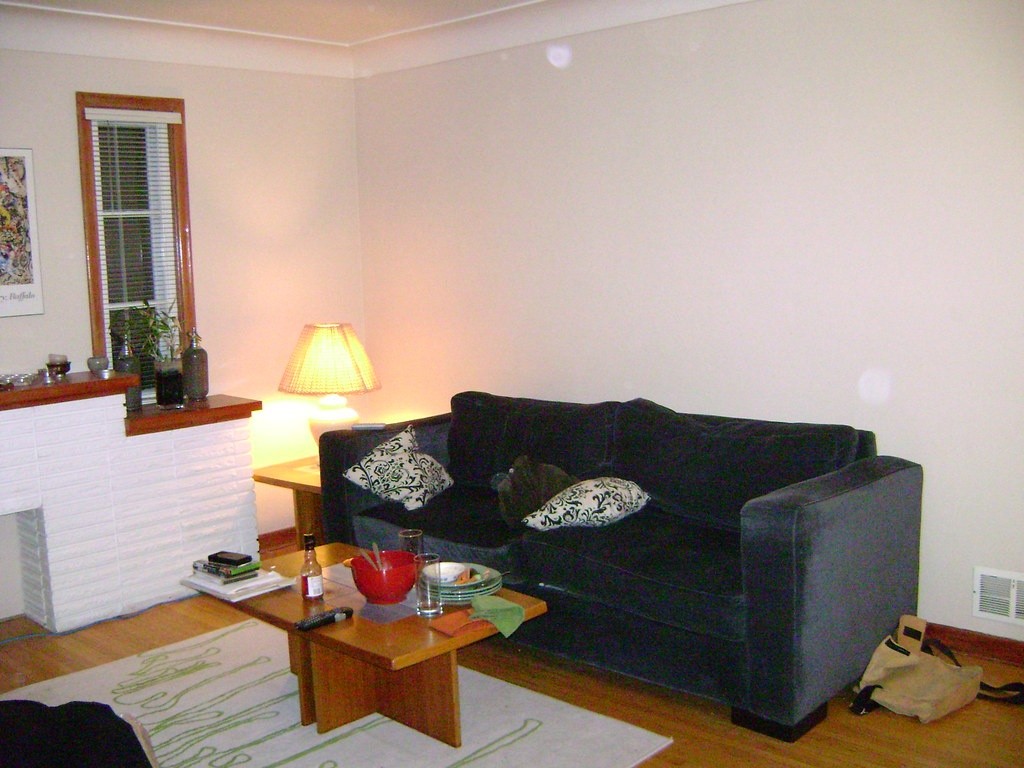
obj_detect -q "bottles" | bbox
[300,533,324,602]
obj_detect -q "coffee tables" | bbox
[195,543,548,747]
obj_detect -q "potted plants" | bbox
[114,298,188,408]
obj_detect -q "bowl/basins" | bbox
[344,550,418,604]
[422,562,464,582]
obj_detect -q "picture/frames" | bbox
[0,146,44,318]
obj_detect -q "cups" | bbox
[414,553,441,620]
[398,529,425,556]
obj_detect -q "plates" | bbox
[417,562,504,606]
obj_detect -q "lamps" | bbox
[278,322,381,445]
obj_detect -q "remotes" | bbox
[295,606,354,631]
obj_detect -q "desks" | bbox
[252,454,325,550]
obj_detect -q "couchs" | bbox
[319,391,925,742]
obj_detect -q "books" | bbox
[194,570,258,585]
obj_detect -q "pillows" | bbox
[500,455,583,530]
[343,423,453,511]
[521,476,651,532]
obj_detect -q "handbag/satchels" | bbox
[848,614,1024,724]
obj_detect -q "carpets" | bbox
[0,619,674,768]
